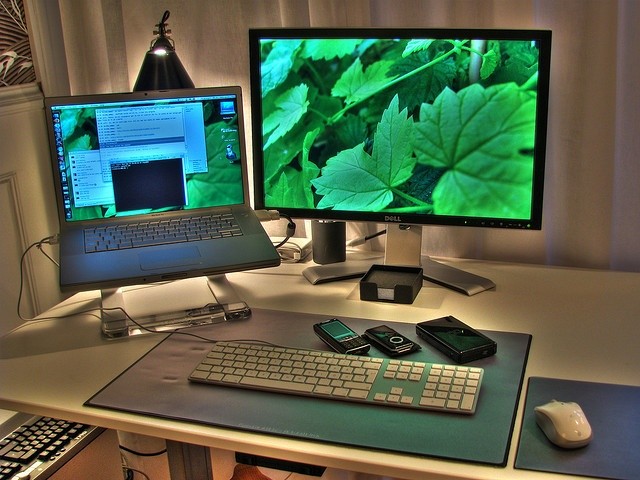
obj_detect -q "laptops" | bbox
[249,27,552,296]
[44,86,281,292]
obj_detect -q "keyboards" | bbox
[0,416,105,480]
[187,341,484,415]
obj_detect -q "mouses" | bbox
[533,399,592,448]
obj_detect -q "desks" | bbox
[0,249,640,480]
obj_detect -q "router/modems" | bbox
[270,237,312,261]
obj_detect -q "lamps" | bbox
[128,9,197,91]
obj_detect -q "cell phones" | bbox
[313,318,371,354]
[361,325,421,357]
[416,316,497,364]
